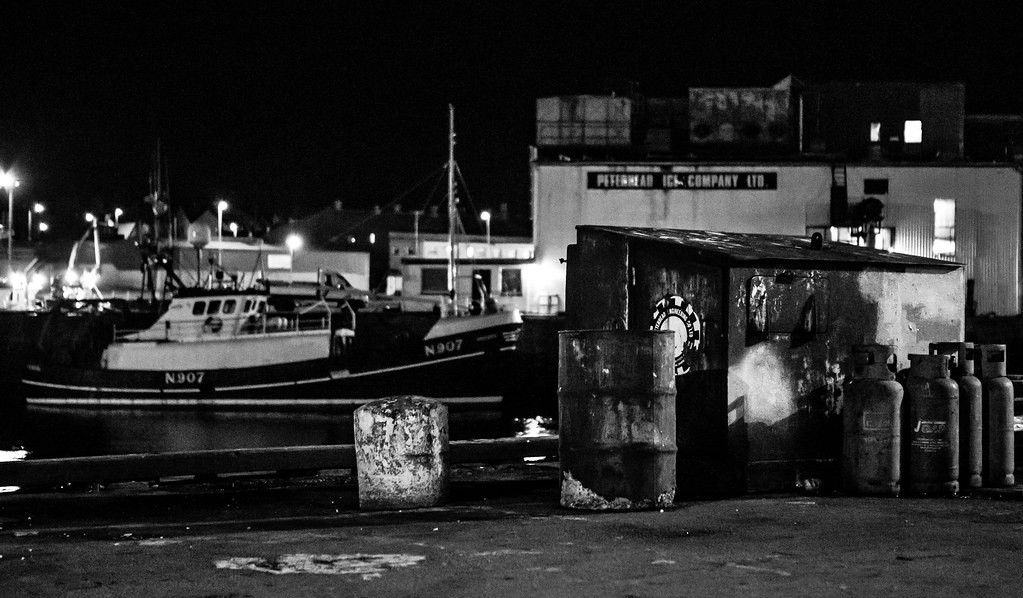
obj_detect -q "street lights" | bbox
[481,211,490,258]
[85,213,100,267]
[217,201,228,267]
[28,204,44,241]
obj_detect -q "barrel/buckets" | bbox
[556,330,677,513]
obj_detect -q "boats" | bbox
[25,224,523,413]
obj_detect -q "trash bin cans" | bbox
[559,329,676,509]
[575,225,967,497]
[522,316,555,363]
